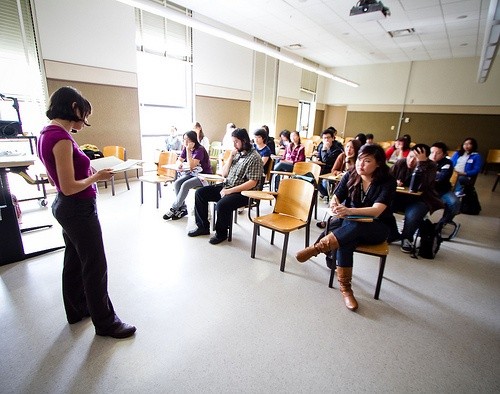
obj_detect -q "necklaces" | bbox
[54,120,72,137]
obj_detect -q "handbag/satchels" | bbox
[411,219,440,259]
[461,183,481,215]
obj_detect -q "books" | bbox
[198,174,224,179]
[90,156,142,173]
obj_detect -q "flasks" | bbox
[409,168,423,193]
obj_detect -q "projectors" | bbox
[350,3,387,21]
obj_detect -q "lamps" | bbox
[118,0,359,87]
[350,0,390,22]
[475,0,500,83]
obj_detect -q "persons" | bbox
[390,142,454,253]
[188,128,263,245]
[163,131,214,220]
[35,87,136,340]
[237,124,306,214]
[295,143,397,311]
[164,125,182,186]
[450,138,483,202]
[221,122,236,151]
[385,134,412,168]
[311,126,374,204]
[190,122,210,154]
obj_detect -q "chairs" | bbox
[103,136,500,299]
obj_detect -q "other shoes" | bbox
[316,222,326,229]
[401,238,412,253]
[188,228,210,237]
[324,196,329,203]
[209,235,227,244]
[97,323,136,339]
[162,208,178,219]
[67,309,90,324]
[172,209,187,220]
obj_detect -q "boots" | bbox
[336,265,359,310]
[296,232,340,263]
